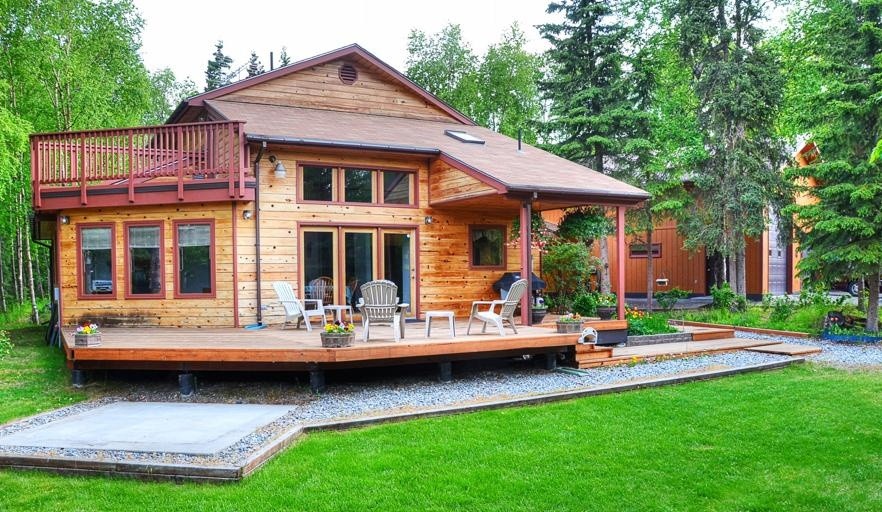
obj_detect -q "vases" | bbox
[555,322,584,333]
[532,312,548,324]
[321,332,355,348]
[75,334,101,346]
[596,308,615,319]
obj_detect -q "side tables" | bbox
[326,303,354,327]
[423,310,457,338]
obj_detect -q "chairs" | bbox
[466,278,528,337]
[272,277,326,328]
[358,280,409,343]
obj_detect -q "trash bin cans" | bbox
[494,272,545,316]
[656,278,669,291]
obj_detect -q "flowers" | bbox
[594,293,615,308]
[557,313,582,322]
[533,300,550,309]
[77,322,100,334]
[325,319,355,333]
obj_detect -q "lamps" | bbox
[269,155,289,180]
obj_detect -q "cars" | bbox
[834,273,882,298]
